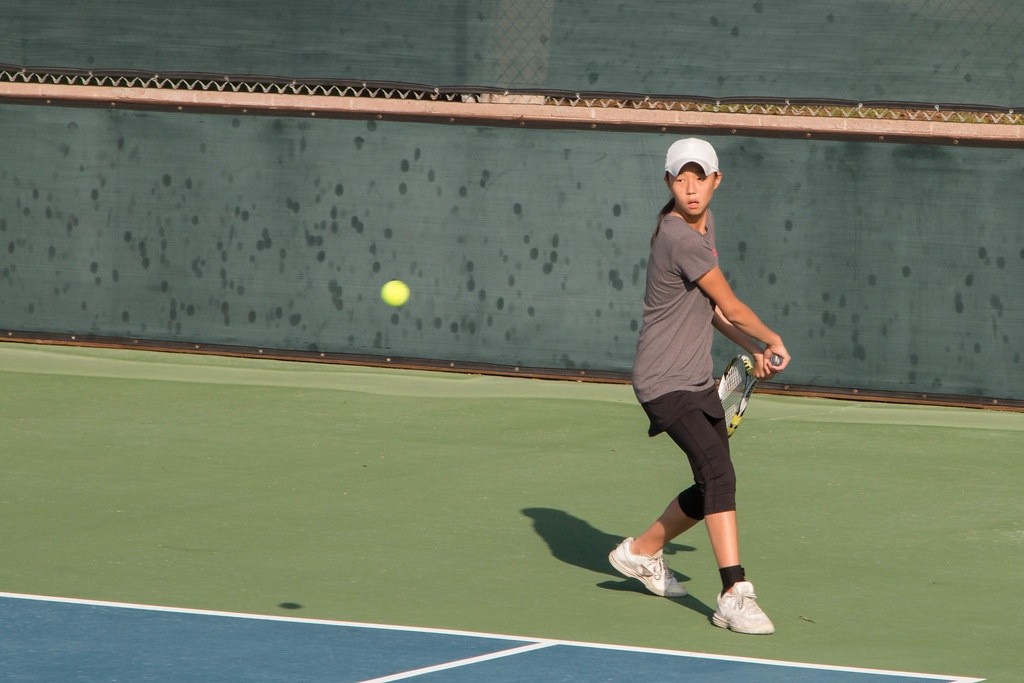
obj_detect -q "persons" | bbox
[608,138,791,636]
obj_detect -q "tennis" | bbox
[381,279,410,306]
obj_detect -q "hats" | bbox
[665,138,719,177]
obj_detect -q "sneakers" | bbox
[712,581,775,634]
[608,536,687,597]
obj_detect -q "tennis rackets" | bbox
[716,352,783,439]
